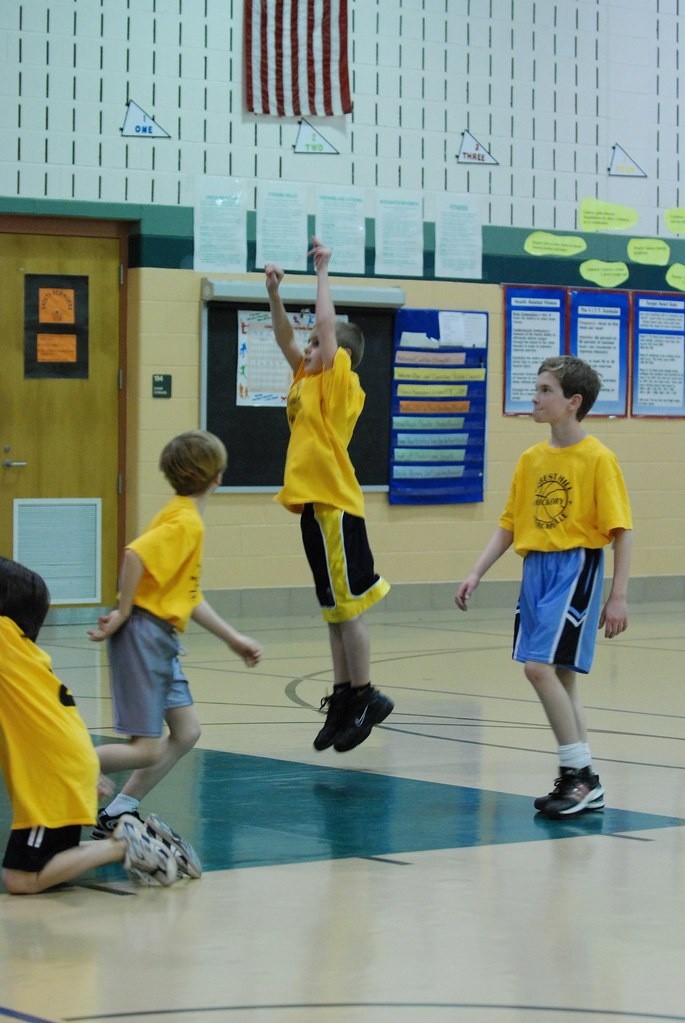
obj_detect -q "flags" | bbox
[242,0,356,118]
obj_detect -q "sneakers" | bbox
[143,813,202,879]
[91,808,145,841]
[112,815,177,887]
[333,690,395,752]
[314,687,345,751]
[534,765,605,816]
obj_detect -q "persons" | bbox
[454,356,637,820]
[264,238,395,753]
[80,428,262,842]
[0,557,202,894]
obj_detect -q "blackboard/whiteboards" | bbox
[199,300,394,490]
